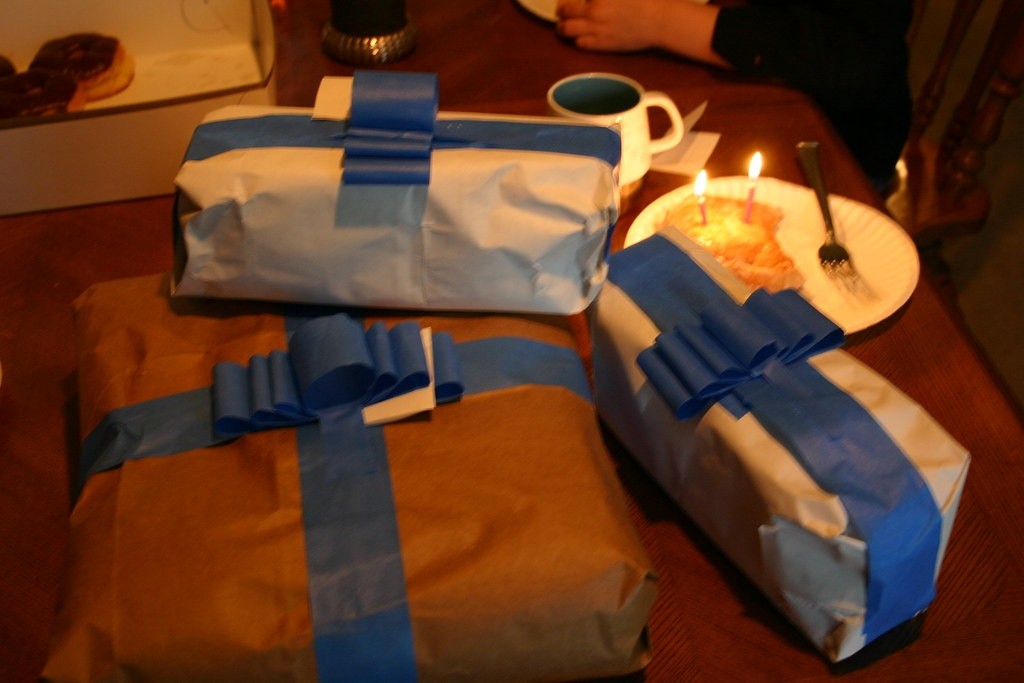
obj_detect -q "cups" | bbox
[545,73,684,185]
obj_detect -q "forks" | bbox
[794,143,857,280]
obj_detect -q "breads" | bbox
[0,31,136,124]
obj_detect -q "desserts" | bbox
[655,195,805,294]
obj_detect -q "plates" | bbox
[623,174,919,343]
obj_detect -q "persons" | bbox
[554,0,915,199]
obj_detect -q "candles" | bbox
[692,168,710,226]
[744,150,763,223]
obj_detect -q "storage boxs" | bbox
[0,0,274,216]
